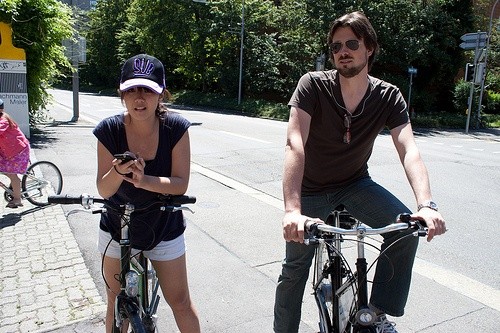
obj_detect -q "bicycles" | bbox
[297,203,449,333]
[1,161,63,206]
[48,191,196,333]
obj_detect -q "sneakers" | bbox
[373,313,398,333]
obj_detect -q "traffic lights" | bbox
[463,62,475,82]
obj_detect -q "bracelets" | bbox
[114,165,129,175]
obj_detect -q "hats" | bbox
[120,54,165,94]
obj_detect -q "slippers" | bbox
[6,202,23,207]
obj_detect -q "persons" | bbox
[0,98,30,207]
[273,12,446,333]
[93,54,200,333]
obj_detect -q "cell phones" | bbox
[114,154,135,164]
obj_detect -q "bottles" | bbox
[125,271,140,297]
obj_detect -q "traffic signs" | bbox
[460,32,487,40]
[459,41,486,51]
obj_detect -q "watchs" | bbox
[417,200,439,212]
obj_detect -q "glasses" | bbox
[329,40,360,54]
